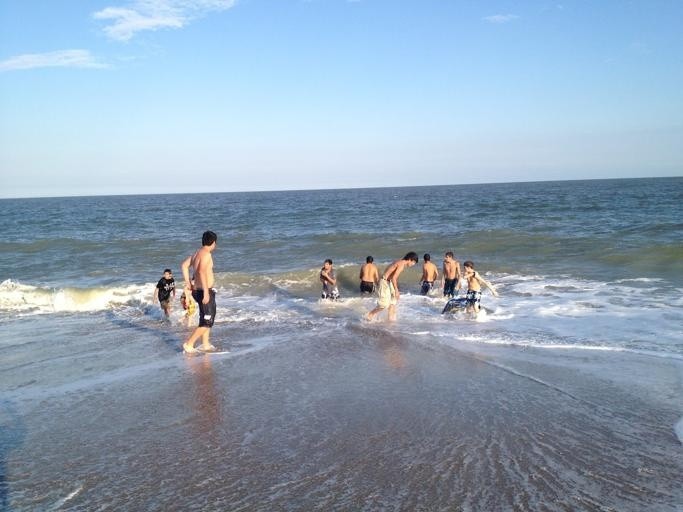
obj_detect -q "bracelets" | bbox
[472,271,475,277]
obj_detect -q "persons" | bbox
[318,258,339,299]
[363,249,419,321]
[181,355,225,443]
[362,329,409,376]
[438,250,462,299]
[179,230,219,354]
[357,255,380,294]
[459,259,500,321]
[152,267,176,319]
[442,296,480,321]
[417,252,438,298]
[180,272,195,320]
